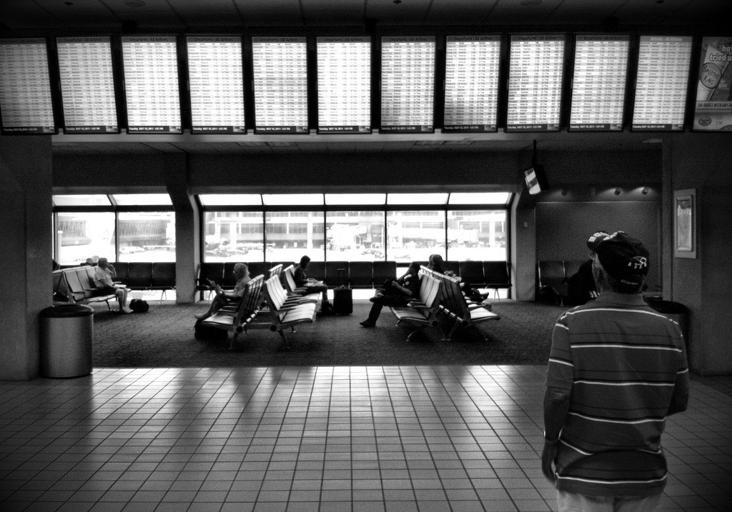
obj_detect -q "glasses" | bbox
[586,250,599,264]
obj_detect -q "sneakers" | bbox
[358,295,388,328]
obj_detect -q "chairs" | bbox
[53,264,133,317]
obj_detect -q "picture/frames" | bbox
[671,187,698,260]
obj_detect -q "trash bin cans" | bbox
[649,302,690,350]
[38,304,94,378]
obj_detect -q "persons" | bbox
[294,255,333,312]
[194,261,253,320]
[430,255,490,303]
[539,229,689,511]
[94,258,134,314]
[360,261,421,327]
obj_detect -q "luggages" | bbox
[332,268,356,315]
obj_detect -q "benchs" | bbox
[111,262,512,303]
[389,265,499,342]
[201,262,324,350]
[539,262,591,305]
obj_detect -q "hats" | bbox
[586,227,653,294]
